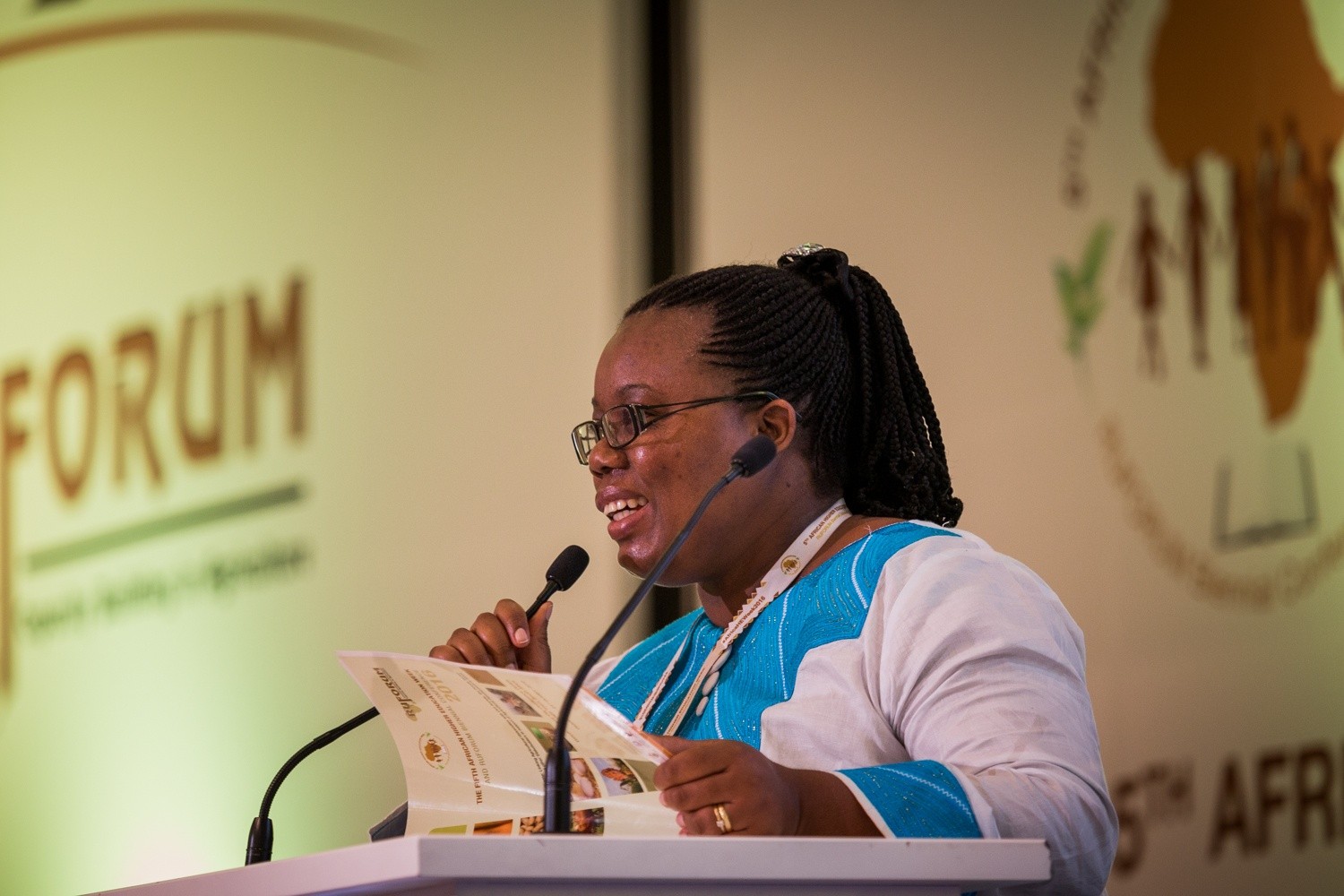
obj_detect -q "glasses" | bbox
[570,391,802,465]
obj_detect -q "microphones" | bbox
[244,543,591,865]
[544,437,786,836]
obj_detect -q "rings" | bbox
[711,803,732,835]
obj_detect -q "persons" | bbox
[431,241,1117,896]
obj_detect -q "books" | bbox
[337,650,684,835]
